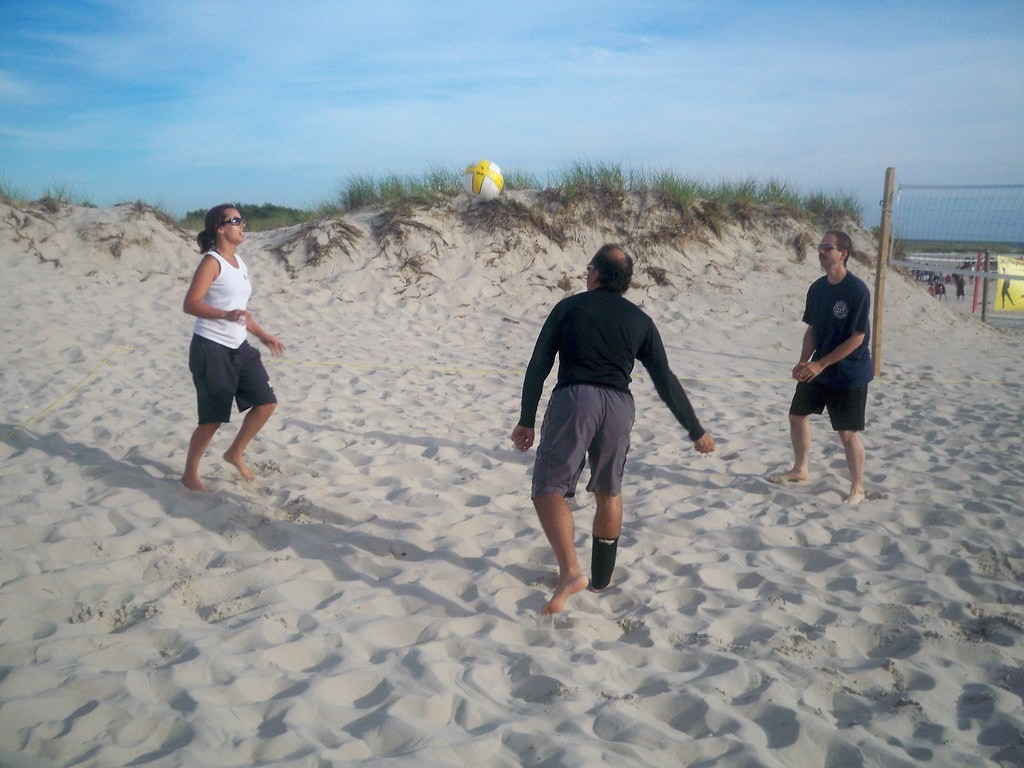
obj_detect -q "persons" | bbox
[512,245,716,616]
[911,256,975,301]
[769,231,874,502]
[180,204,286,491]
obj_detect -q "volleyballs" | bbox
[461,158,505,199]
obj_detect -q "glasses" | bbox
[587,263,593,270]
[220,217,243,226]
[818,244,842,250]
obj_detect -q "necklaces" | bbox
[212,250,222,256]
